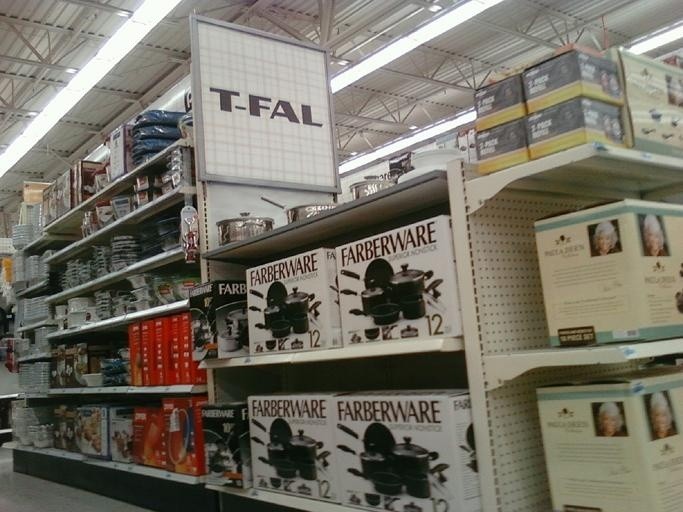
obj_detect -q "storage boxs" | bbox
[336,214,463,347]
[109,419,133,463]
[473,46,683,175]
[247,391,341,504]
[110,124,133,180]
[536,366,683,512]
[246,247,343,353]
[76,403,132,460]
[161,397,207,477]
[202,401,253,490]
[189,280,247,360]
[133,405,167,469]
[533,198,683,347]
[334,390,482,511]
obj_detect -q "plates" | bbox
[410,149,463,169]
[12,224,55,448]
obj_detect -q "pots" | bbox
[250,417,330,481]
[249,280,322,340]
[217,308,248,351]
[336,423,449,500]
[216,212,275,247]
[341,259,443,326]
[260,196,340,224]
[349,180,394,200]
[460,423,478,473]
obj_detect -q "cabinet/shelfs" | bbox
[447,141,683,512]
[44,127,219,512]
[201,169,486,512]
[0,230,77,482]
